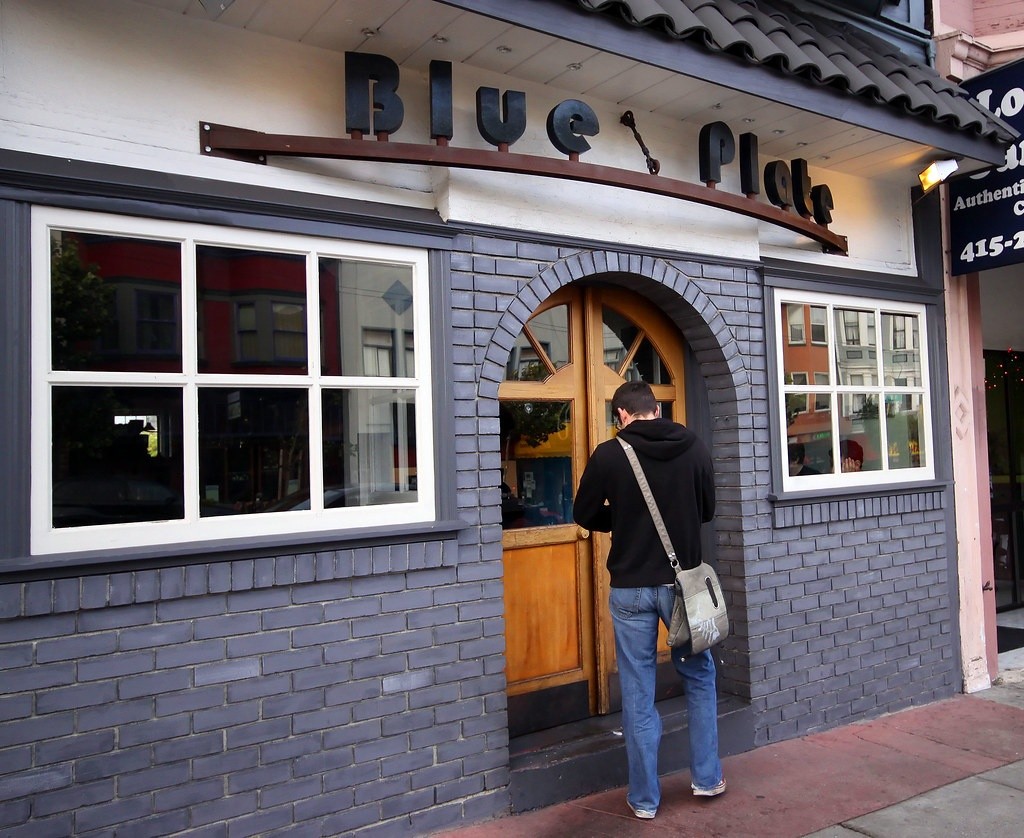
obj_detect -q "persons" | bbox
[788,435,820,476]
[572,381,727,819]
[829,440,863,474]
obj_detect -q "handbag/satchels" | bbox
[665,563,730,655]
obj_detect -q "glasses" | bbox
[615,415,624,430]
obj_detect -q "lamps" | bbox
[918,157,960,194]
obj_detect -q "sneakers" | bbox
[692,777,728,796]
[626,792,657,819]
